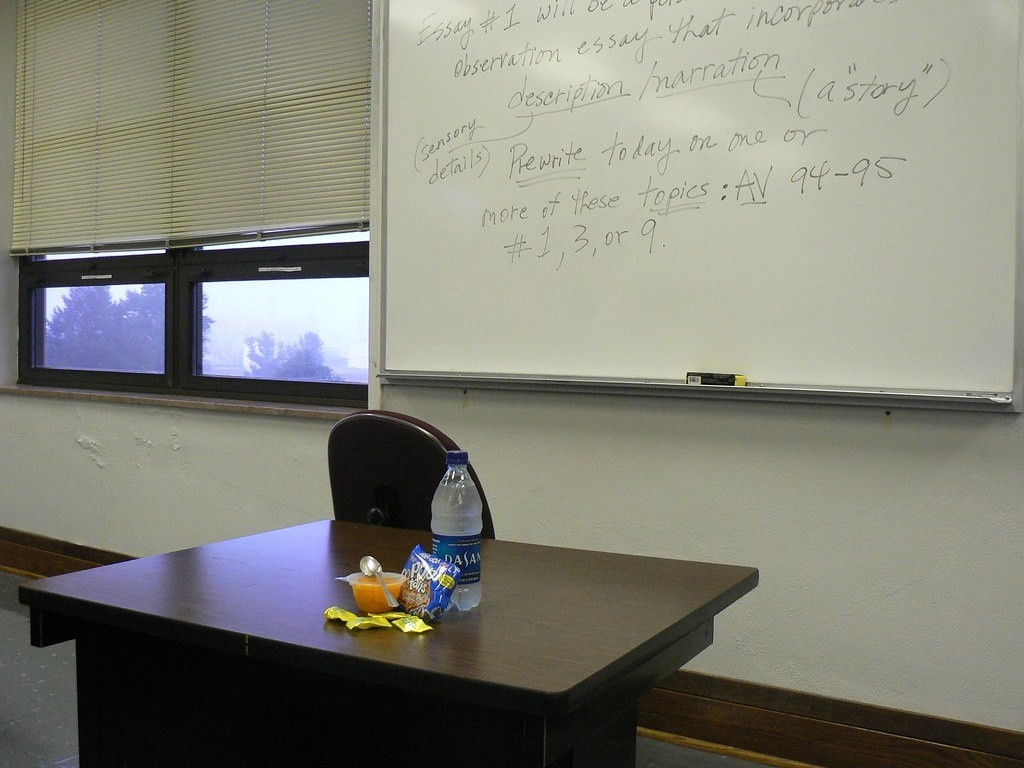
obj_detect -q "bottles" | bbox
[430,450,483,612]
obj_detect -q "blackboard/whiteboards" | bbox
[378,0,1022,413]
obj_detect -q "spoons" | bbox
[359,555,400,608]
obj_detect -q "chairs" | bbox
[325,409,495,537]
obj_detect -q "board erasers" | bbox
[685,371,746,388]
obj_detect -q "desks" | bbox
[17,518,759,767]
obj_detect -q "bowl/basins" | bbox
[345,571,406,614]
